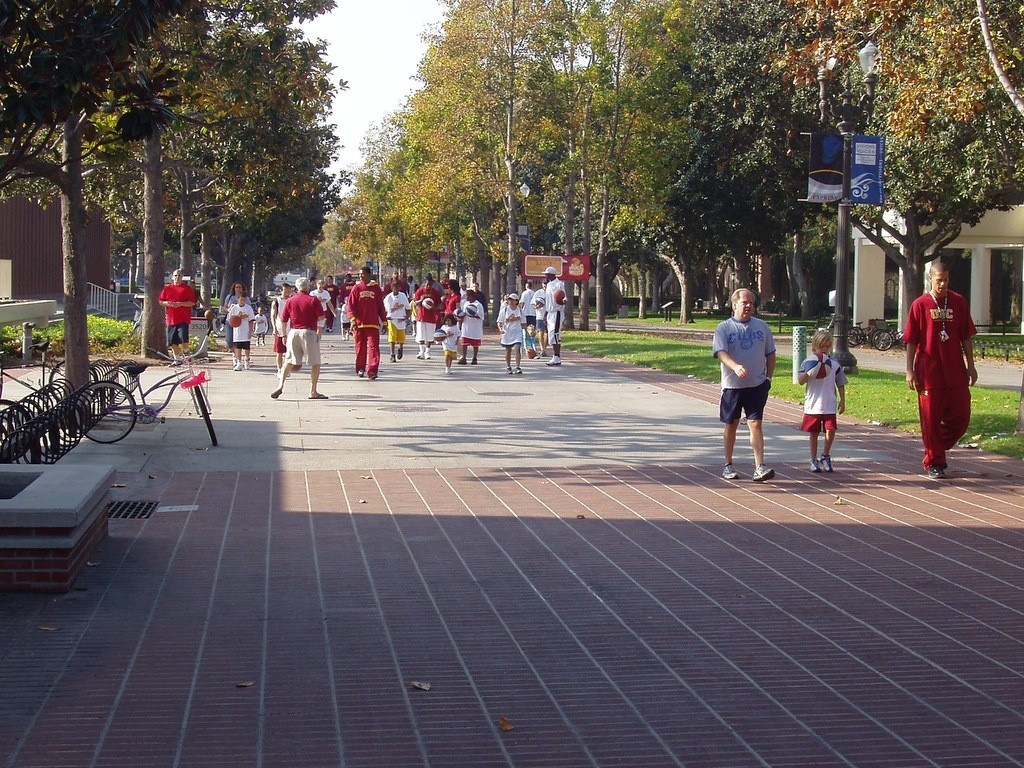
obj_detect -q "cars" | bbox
[163,268,221,293]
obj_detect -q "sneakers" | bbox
[928,464,945,478]
[752,464,775,480]
[357,346,561,379]
[809,459,821,472]
[722,464,740,479]
[820,455,833,471]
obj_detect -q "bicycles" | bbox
[810,313,908,351]
[0,340,99,410]
[125,298,168,345]
[74,326,219,447]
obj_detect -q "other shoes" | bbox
[168,360,182,366]
[232,358,250,370]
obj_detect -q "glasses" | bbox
[544,282,547,284]
[173,274,181,277]
[235,287,241,288]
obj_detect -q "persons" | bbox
[712,288,776,482]
[224,281,269,372]
[384,270,487,375]
[496,266,567,374]
[158,269,196,365]
[189,281,209,318]
[798,330,849,471]
[902,263,978,478]
[271,265,388,399]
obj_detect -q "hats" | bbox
[295,277,312,291]
[542,278,549,282]
[508,293,520,301]
[542,267,556,274]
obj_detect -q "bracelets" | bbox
[766,377,771,382]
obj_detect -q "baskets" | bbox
[173,357,211,389]
[133,310,140,321]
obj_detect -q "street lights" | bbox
[817,38,884,377]
[514,182,530,291]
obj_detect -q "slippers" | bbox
[270,387,282,399]
[308,393,328,399]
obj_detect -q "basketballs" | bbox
[422,298,434,309]
[434,329,446,341]
[204,310,213,319]
[554,289,565,301]
[453,309,464,320]
[230,315,242,327]
[465,304,477,316]
[536,296,546,307]
[527,349,536,359]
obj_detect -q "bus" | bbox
[324,272,363,290]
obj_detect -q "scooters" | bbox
[209,292,270,338]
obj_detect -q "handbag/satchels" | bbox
[221,316,226,324]
